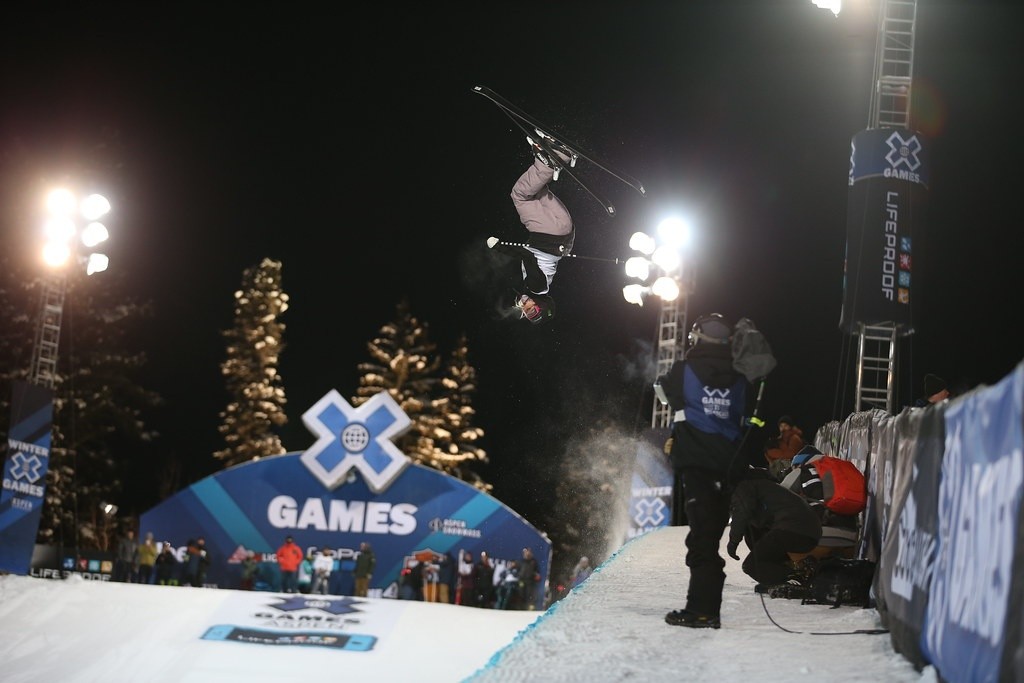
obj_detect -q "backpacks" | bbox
[803,455,866,516]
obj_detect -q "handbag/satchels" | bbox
[731,317,778,383]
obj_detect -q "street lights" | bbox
[622,214,695,442]
[34,181,111,574]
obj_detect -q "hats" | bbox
[778,415,795,427]
[925,374,949,397]
[790,444,823,466]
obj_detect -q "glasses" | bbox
[524,304,536,316]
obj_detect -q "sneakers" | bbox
[534,129,579,167]
[526,136,563,181]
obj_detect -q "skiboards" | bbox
[470,81,650,219]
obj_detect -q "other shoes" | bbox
[754,583,769,593]
[665,609,722,630]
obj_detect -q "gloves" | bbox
[726,540,740,561]
[487,236,499,249]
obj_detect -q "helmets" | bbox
[687,313,733,346]
[515,291,556,328]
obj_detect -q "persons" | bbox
[572,557,593,586]
[352,543,376,598]
[915,374,950,408]
[487,129,576,326]
[400,547,541,610]
[654,313,757,629]
[239,554,261,591]
[117,529,210,587]
[277,536,333,596]
[727,416,860,594]
